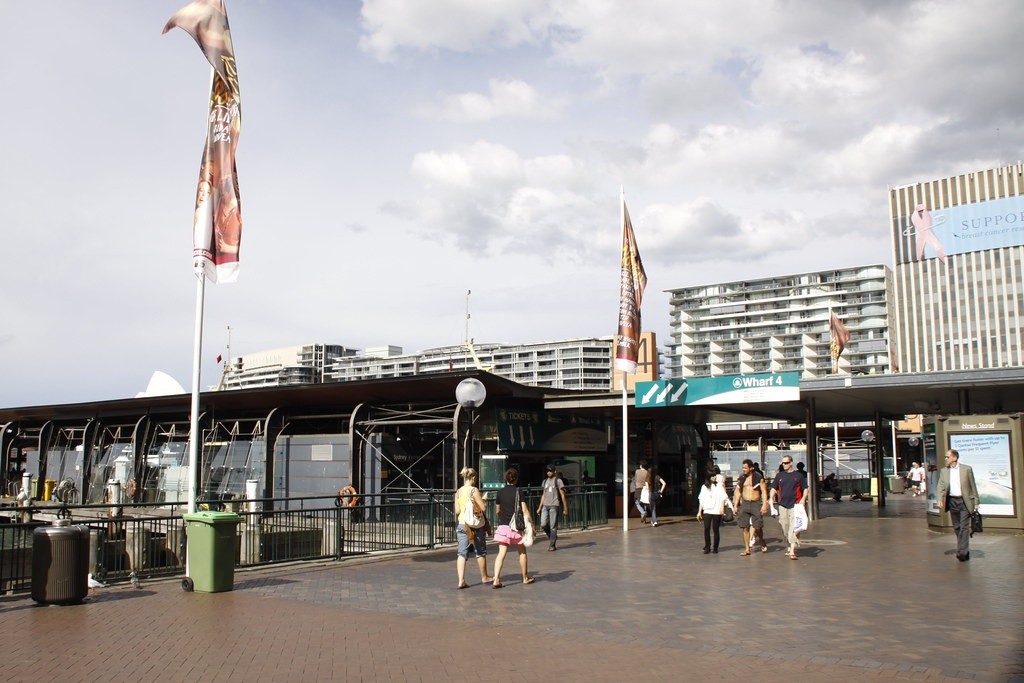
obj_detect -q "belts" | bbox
[950,496,963,500]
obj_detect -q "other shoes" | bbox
[482,578,494,584]
[702,547,711,554]
[917,491,920,496]
[547,546,557,551]
[956,551,970,561]
[640,513,648,523]
[523,576,535,584]
[456,583,470,589]
[492,583,505,589]
[740,551,751,556]
[712,548,719,553]
[761,546,768,552]
[651,521,658,527]
[912,494,916,497]
[749,540,754,548]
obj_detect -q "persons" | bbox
[935,450,980,562]
[734,462,765,548]
[734,459,768,555]
[493,468,534,586]
[705,460,725,526]
[627,460,666,527]
[769,455,809,559]
[454,466,494,587]
[583,471,594,483]
[696,468,733,554]
[778,462,807,478]
[537,464,568,551]
[824,473,841,502]
[907,461,929,497]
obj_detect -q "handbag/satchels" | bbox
[514,487,525,531]
[920,481,926,493]
[969,510,983,538]
[465,487,485,528]
[794,504,808,533]
[639,486,651,504]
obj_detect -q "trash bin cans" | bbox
[887,475,898,491]
[30,518,90,604]
[181,512,242,593]
[891,476,905,494]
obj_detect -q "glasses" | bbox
[781,461,791,464]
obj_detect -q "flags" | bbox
[830,311,851,374]
[616,202,647,375]
[160,0,242,279]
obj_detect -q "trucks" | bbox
[882,457,909,477]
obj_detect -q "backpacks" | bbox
[544,477,566,506]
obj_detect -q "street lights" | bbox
[908,437,923,467]
[455,378,486,468]
[862,430,874,502]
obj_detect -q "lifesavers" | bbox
[125,479,137,500]
[339,485,358,507]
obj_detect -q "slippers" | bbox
[785,551,798,560]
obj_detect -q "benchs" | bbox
[820,490,835,498]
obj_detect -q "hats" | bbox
[545,464,556,471]
